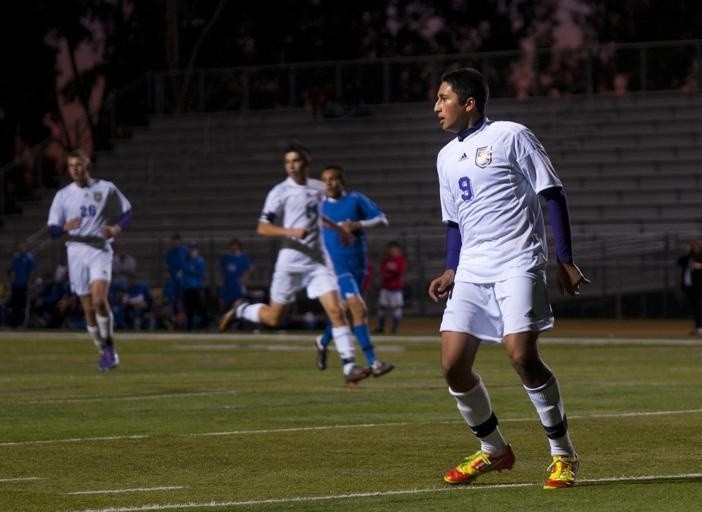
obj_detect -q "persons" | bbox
[676,240,701,337]
[427,67,591,490]
[312,164,394,379]
[46,148,134,373]
[217,139,372,389]
[682,241,695,293]
[4,233,408,336]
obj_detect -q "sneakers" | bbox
[369,358,396,378]
[96,344,121,372]
[342,362,373,384]
[314,334,328,370]
[539,451,580,490]
[443,440,516,486]
[218,297,248,331]
